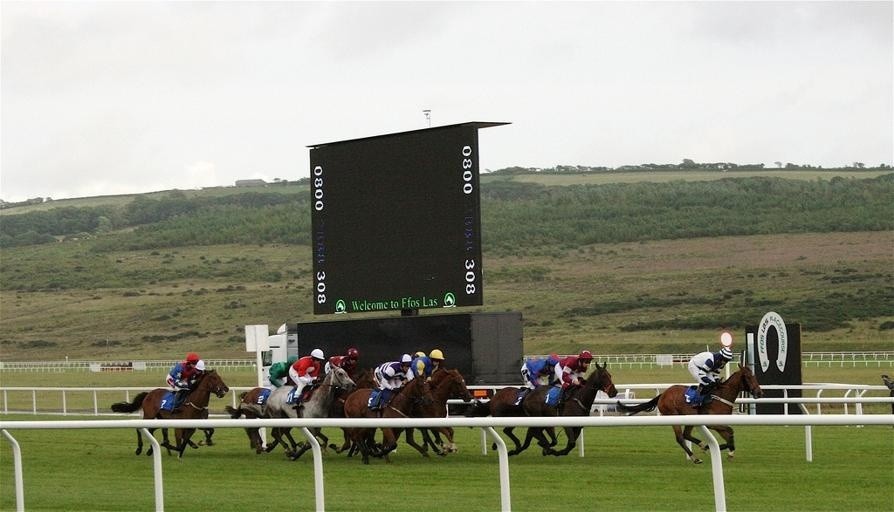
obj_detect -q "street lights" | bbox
[422,109,433,129]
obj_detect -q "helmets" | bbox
[719,346,733,359]
[399,353,412,362]
[287,355,297,364]
[578,350,593,359]
[428,349,445,360]
[548,354,559,365]
[414,351,425,357]
[194,359,204,370]
[187,354,198,360]
[310,349,325,360]
[347,348,359,359]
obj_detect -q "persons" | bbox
[371,353,412,409]
[193,360,204,374]
[687,346,734,408]
[554,350,593,389]
[324,345,359,374]
[164,353,203,408]
[406,351,427,382]
[513,353,559,403]
[266,355,297,391]
[288,348,324,401]
[408,349,444,382]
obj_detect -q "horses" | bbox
[111,368,225,458]
[226,361,473,465]
[524,361,618,457]
[467,377,561,456]
[616,363,765,465]
[158,369,229,449]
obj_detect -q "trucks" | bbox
[256,311,525,416]
